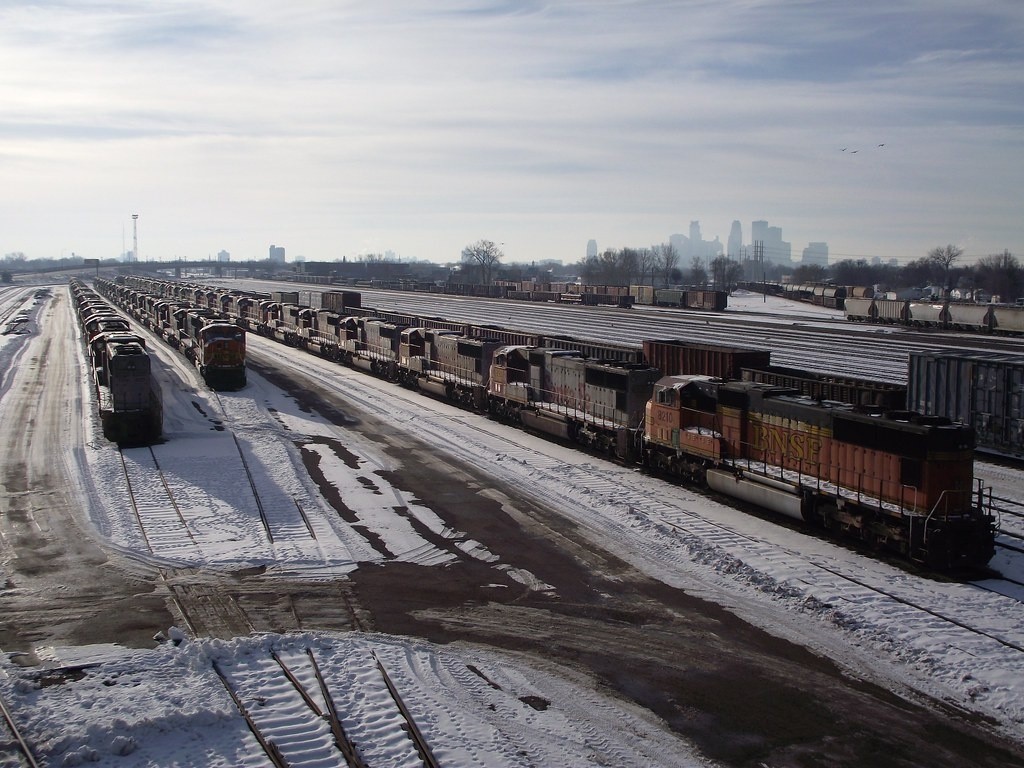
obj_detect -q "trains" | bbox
[107,272,1024,582]
[93,274,249,394]
[67,275,163,450]
